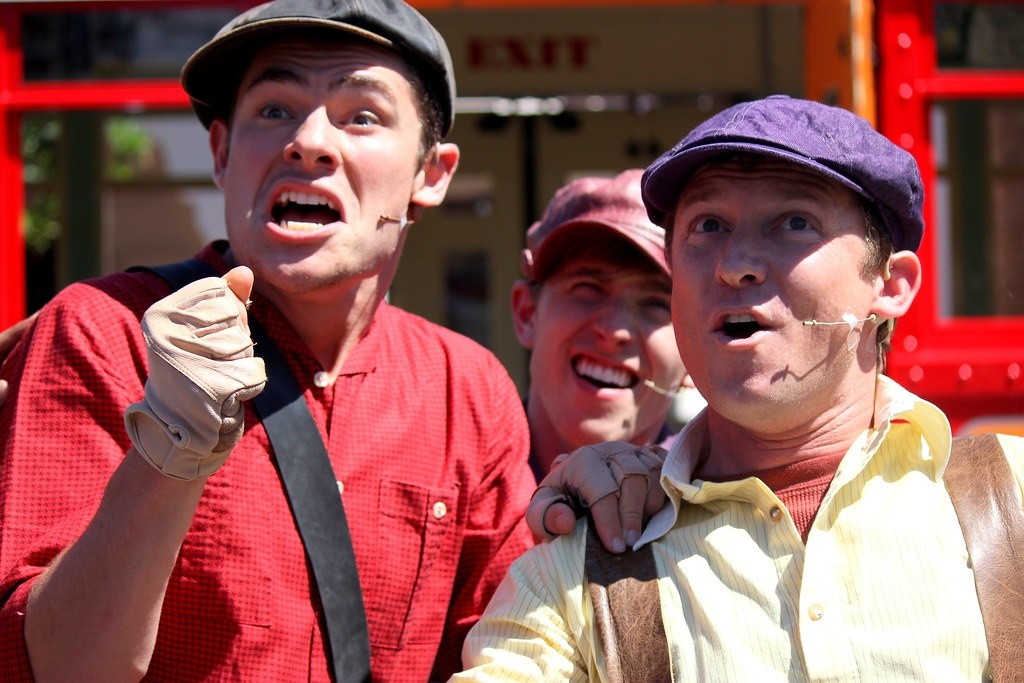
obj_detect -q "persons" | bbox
[0,0,673,683]
[443,94,1024,682]
[512,169,696,485]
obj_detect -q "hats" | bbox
[520,170,673,295]
[180,0,456,140]
[641,94,922,260]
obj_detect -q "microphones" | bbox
[644,380,682,396]
[379,215,418,226]
[802,314,877,327]
